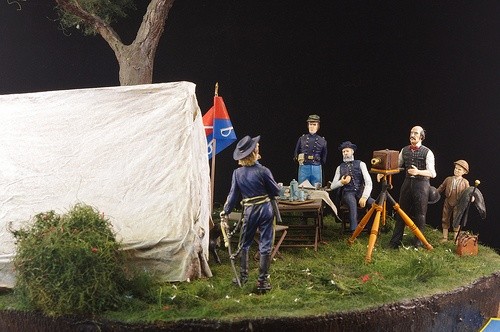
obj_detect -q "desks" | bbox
[276,184,325,252]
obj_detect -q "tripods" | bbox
[348,175,434,261]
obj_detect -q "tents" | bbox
[0,81,212,291]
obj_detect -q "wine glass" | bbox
[299,154,304,166]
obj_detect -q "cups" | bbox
[315,182,321,197]
[298,190,306,201]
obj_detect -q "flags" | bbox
[201,94,237,160]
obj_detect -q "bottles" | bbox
[290,179,298,200]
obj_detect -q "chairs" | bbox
[340,198,371,235]
[229,212,289,263]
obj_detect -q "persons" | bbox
[436,160,475,245]
[330,141,381,238]
[292,115,326,189]
[220,135,282,290]
[389,126,436,250]
